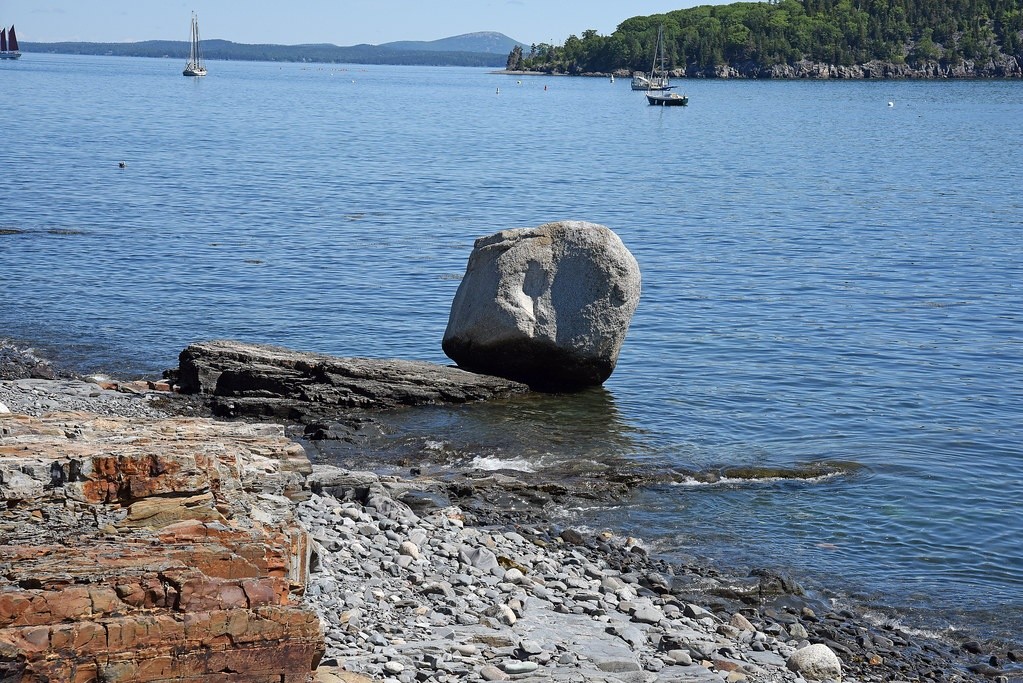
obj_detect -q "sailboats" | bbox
[1,25,22,59]
[631,26,690,108]
[184,9,208,77]
